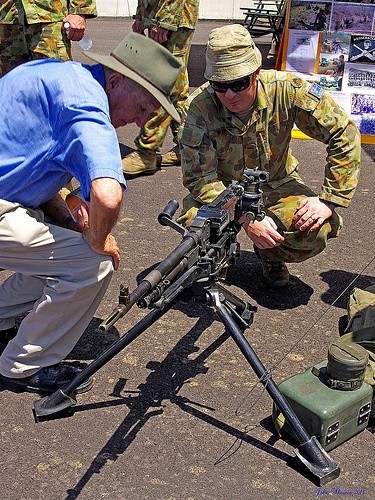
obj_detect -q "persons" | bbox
[175,23,361,287]
[122,0,200,176]
[0,0,98,201]
[351,93,375,115]
[333,55,345,76]
[0,32,182,394]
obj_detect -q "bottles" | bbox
[64,22,94,51]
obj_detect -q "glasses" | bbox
[209,74,251,93]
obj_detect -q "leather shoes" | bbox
[0,361,94,393]
[0,325,19,347]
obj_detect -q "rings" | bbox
[309,216,316,222]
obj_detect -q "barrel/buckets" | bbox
[65,186,92,225]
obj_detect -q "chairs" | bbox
[240,0,288,49]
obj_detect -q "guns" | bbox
[31,167,341,487]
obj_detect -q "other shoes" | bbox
[121,151,157,175]
[253,243,289,286]
[161,145,181,166]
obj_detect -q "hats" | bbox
[204,24,262,81]
[82,32,183,124]
